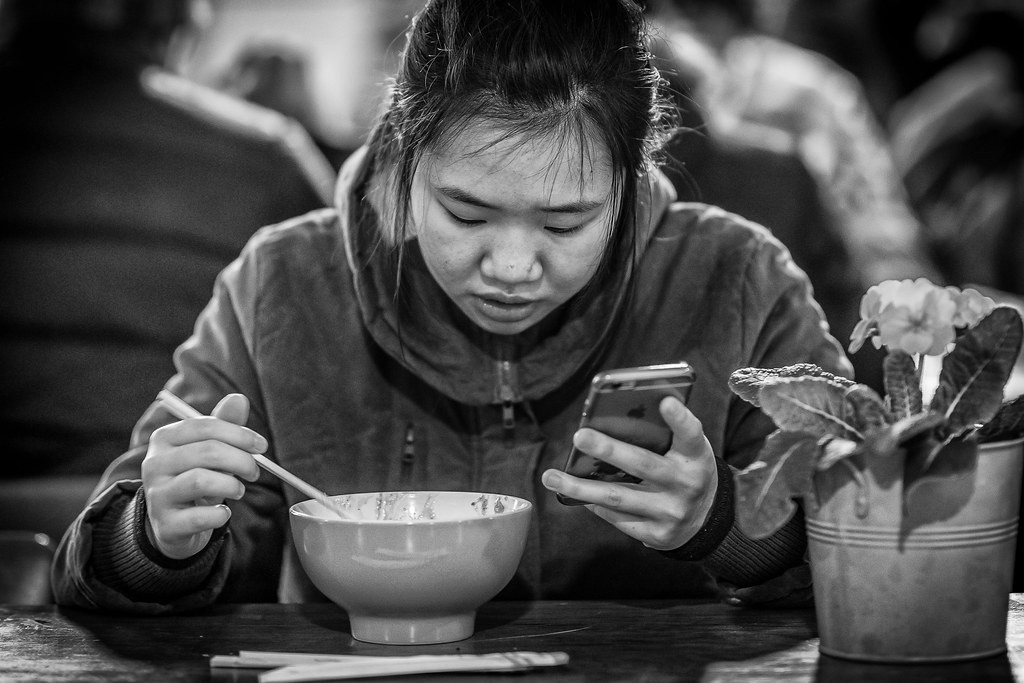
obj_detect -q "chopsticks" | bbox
[211,650,568,683]
[157,387,360,521]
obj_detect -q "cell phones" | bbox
[560,363,695,509]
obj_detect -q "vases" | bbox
[801,433,1022,663]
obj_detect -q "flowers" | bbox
[723,276,1024,543]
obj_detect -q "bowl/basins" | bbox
[290,490,534,645]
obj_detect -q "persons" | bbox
[0,2,1023,663]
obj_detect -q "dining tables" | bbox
[0,591,1023,683]
